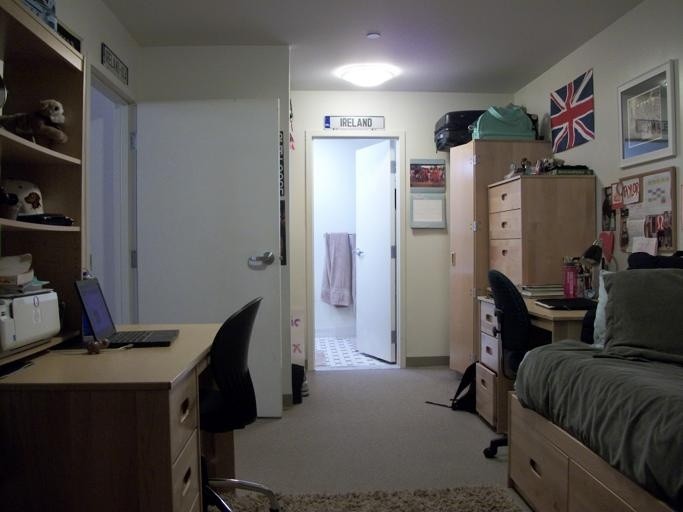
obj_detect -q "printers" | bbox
[0,287,62,351]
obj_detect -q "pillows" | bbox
[592,267,682,362]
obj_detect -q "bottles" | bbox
[561,262,578,299]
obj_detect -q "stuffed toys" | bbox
[34,99,69,153]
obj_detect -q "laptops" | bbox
[75,277,180,347]
[533,297,598,310]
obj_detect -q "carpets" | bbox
[204,486,526,511]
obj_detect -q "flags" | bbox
[549,67,595,154]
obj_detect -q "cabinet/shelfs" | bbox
[444,139,597,375]
[0,1,88,367]
[0,317,235,511]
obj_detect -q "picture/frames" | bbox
[616,59,677,169]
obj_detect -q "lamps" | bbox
[582,240,601,266]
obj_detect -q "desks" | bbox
[473,293,598,437]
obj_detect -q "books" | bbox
[520,284,566,296]
[543,169,585,176]
[0,253,44,293]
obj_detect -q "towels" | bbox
[319,231,355,308]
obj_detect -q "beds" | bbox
[504,338,682,511]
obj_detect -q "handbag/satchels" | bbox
[472,102,536,140]
[451,360,480,412]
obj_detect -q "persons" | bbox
[620,221,628,250]
[410,165,444,182]
[656,211,672,249]
[600,187,615,231]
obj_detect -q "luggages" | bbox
[434,110,538,151]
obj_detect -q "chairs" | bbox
[200,295,280,511]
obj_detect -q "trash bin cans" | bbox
[292,363,304,404]
